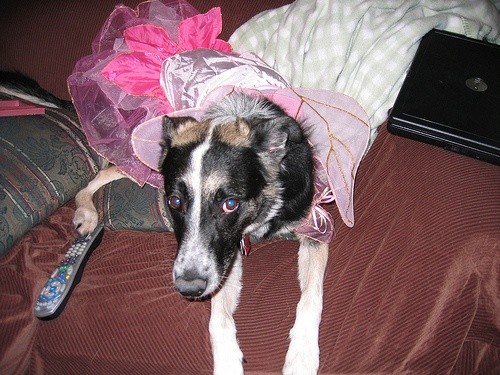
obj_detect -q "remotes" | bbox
[34,226,104,321]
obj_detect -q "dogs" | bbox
[72,0,334,375]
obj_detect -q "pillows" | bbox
[0,69,143,258]
[92,161,176,232]
